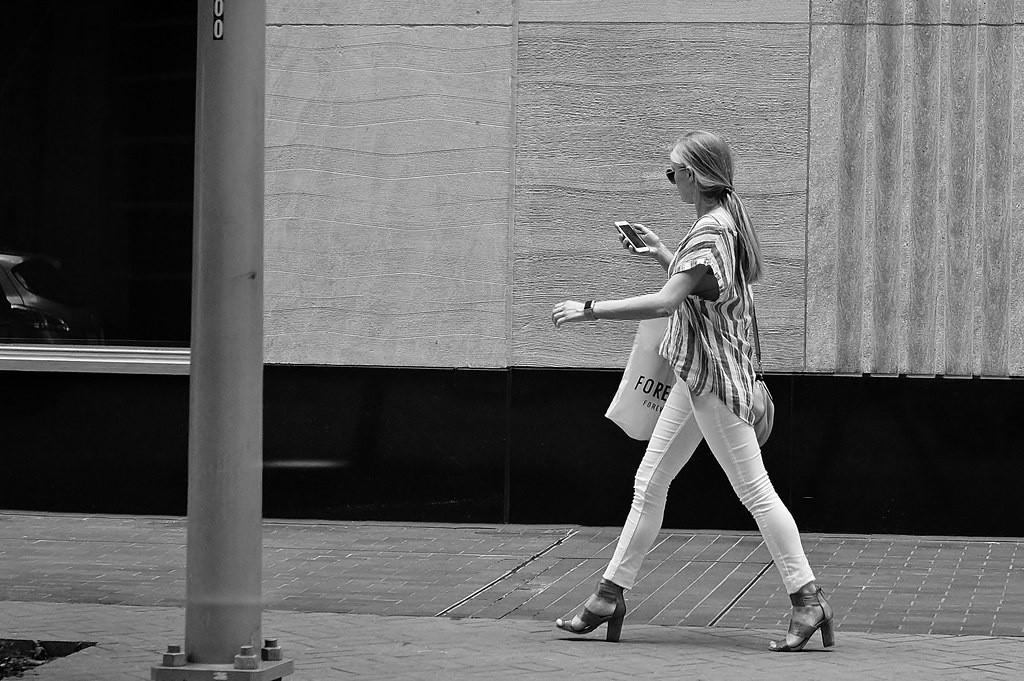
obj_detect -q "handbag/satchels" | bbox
[604,319,676,442]
[752,379,776,448]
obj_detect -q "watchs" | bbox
[584,300,597,321]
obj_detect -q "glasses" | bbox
[666,167,687,184]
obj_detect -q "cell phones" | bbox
[615,220,650,253]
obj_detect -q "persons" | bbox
[551,130,835,652]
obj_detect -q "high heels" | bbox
[769,587,835,652]
[555,581,627,642]
